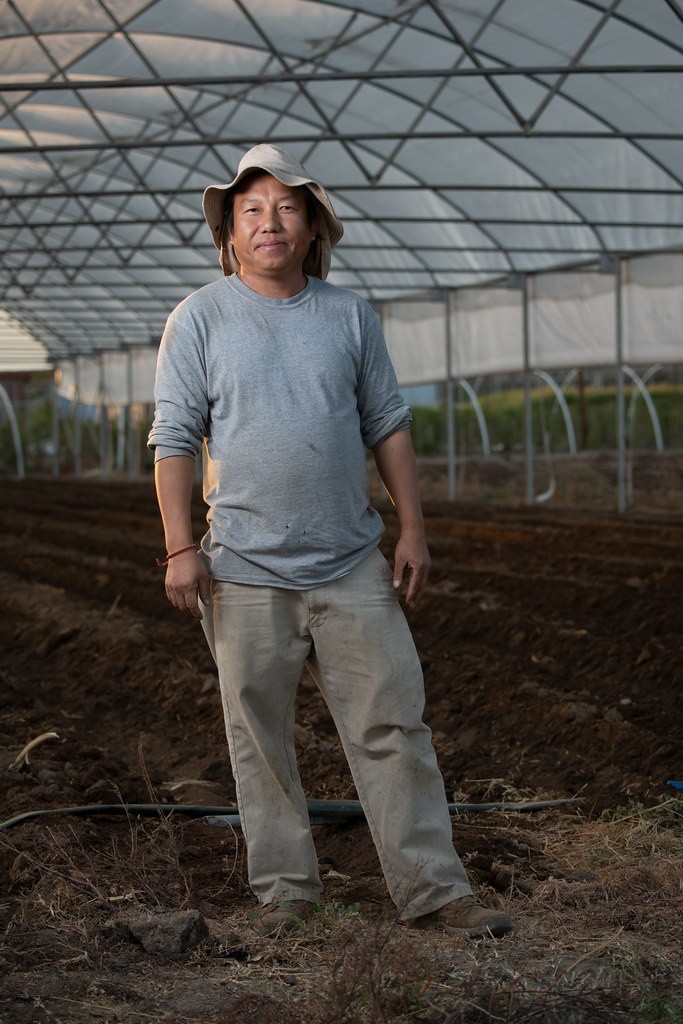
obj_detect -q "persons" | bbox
[148,143,513,939]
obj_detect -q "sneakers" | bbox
[259,901,313,932]
[408,894,511,937]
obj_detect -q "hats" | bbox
[202,144,345,252]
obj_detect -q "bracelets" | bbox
[156,544,197,565]
